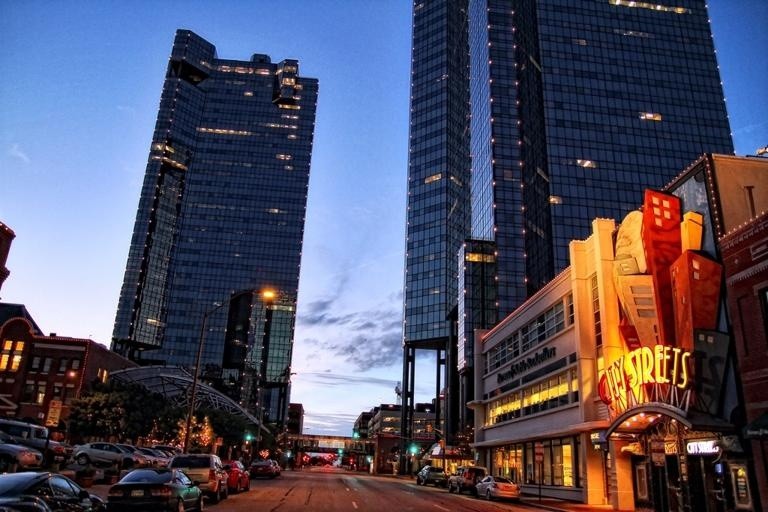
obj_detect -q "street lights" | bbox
[182,286,277,452]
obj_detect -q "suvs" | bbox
[446,465,487,494]
[168,454,230,503]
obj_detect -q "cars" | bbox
[0,469,106,512]
[470,474,522,501]
[106,465,205,511]
[0,416,281,495]
[415,465,445,486]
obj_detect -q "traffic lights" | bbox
[410,446,416,455]
[353,431,369,440]
